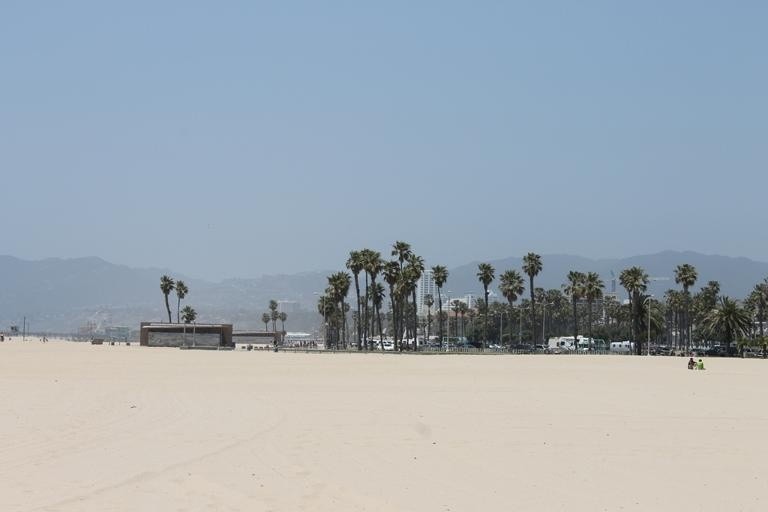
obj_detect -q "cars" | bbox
[508,336,635,355]
[351,335,477,352]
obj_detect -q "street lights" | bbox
[514,306,530,346]
[446,290,452,352]
[313,290,334,349]
[484,289,489,349]
[647,296,655,356]
[494,310,507,347]
[535,301,555,347]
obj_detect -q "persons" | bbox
[688,357,696,369]
[695,359,704,370]
